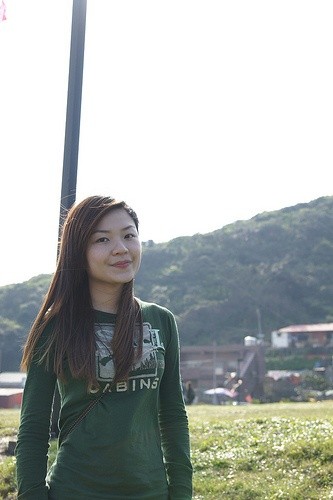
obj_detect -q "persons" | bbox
[179,378,245,405]
[13,192,195,500]
